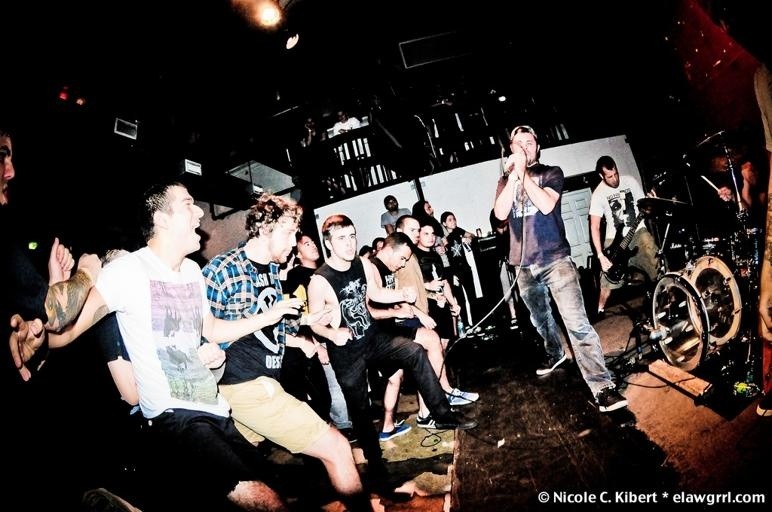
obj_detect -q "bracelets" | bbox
[508,172,520,183]
[597,251,604,258]
[308,128,313,133]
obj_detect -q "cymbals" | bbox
[693,130,725,146]
[637,196,691,213]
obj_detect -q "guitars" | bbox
[602,171,670,285]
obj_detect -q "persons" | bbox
[719,161,767,210]
[587,154,669,317]
[757,61,772,417]
[493,125,629,413]
[201,166,489,512]
[7,181,308,511]
[332,107,369,192]
[84,248,227,408]
[302,115,330,147]
[0,126,102,335]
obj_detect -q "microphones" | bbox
[505,164,515,178]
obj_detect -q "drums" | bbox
[701,237,728,260]
[729,228,765,268]
[652,254,742,370]
[662,229,696,274]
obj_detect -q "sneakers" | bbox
[338,388,480,444]
[457,318,519,339]
[598,386,628,413]
[536,350,567,376]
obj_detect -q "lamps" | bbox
[253,5,302,52]
